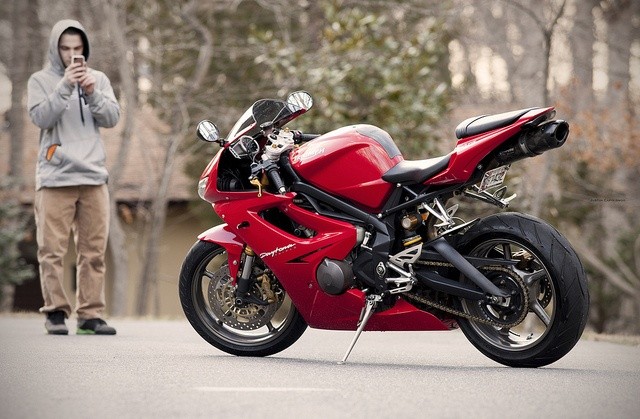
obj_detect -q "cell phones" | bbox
[71,55,84,78]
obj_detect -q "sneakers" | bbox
[76,323,117,335]
[44,316,69,335]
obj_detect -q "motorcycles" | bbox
[177,89,592,370]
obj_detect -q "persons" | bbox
[27,18,120,335]
